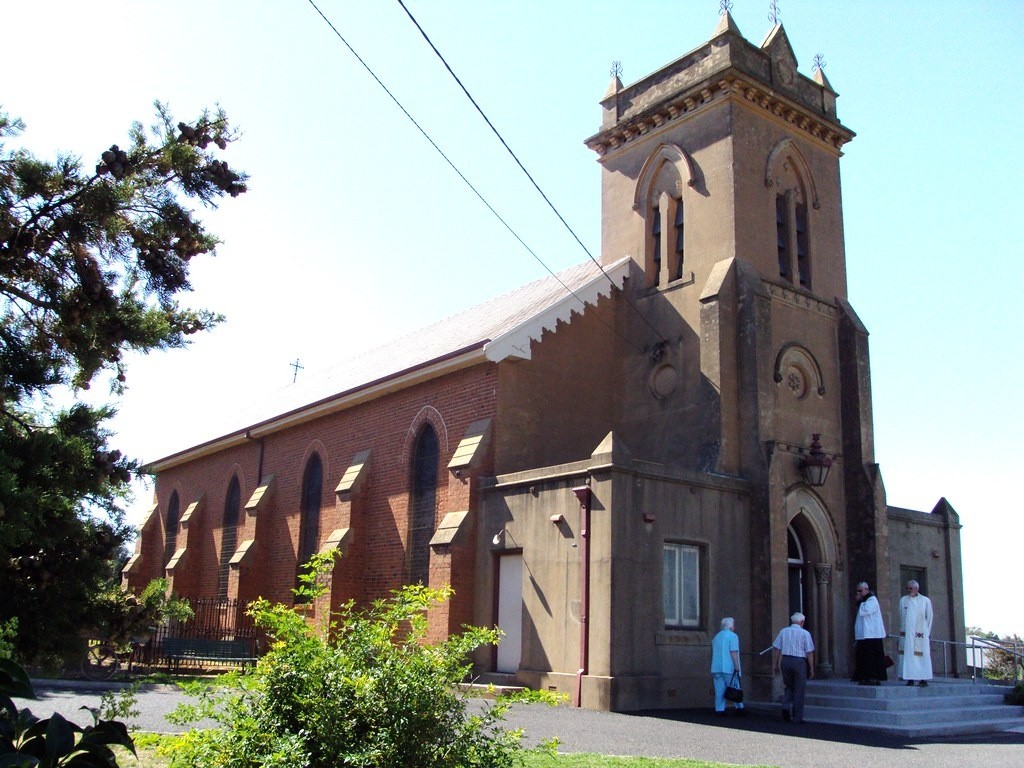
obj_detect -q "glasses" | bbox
[906,587,913,590]
[857,589,862,592]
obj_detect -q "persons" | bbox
[708,617,744,711]
[772,612,815,724]
[854,580,888,685]
[896,579,934,687]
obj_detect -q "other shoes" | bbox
[906,680,928,687]
[782,709,806,724]
[858,678,880,685]
[717,709,747,718]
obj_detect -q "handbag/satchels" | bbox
[885,655,895,669]
[723,669,745,703]
